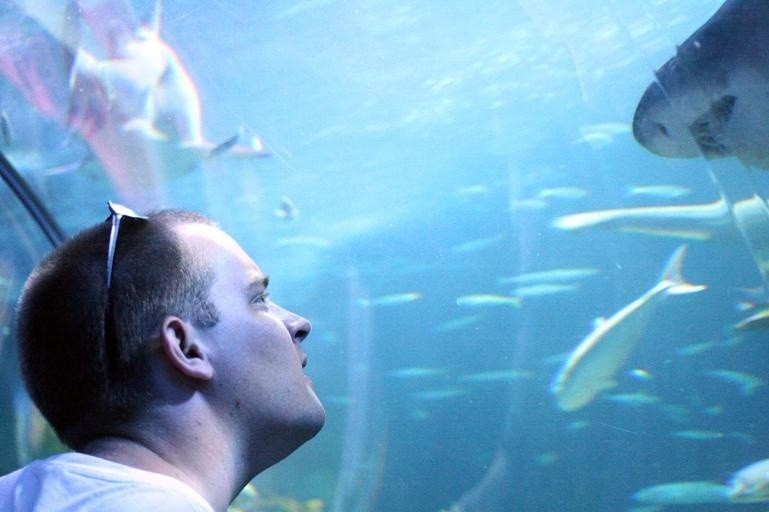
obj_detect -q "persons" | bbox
[0,203,327,511]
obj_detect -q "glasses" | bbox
[99,198,149,364]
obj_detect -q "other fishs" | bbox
[329,1,767,512]
